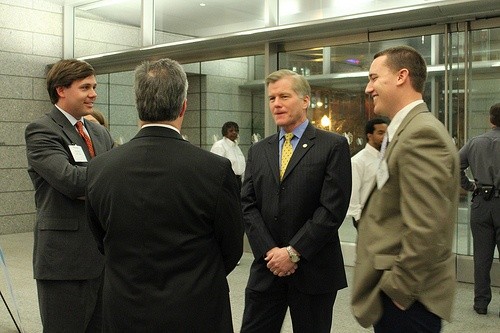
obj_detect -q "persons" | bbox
[234,68,352,333]
[24,54,121,333]
[458,101,500,317]
[351,45,462,333]
[209,119,247,198]
[83,57,244,333]
[349,119,390,233]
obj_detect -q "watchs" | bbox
[286,246,302,263]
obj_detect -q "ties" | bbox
[75,122,95,160]
[377,131,388,172]
[280,133,294,182]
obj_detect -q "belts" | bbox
[476,189,500,197]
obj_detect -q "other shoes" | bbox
[474,305,487,314]
